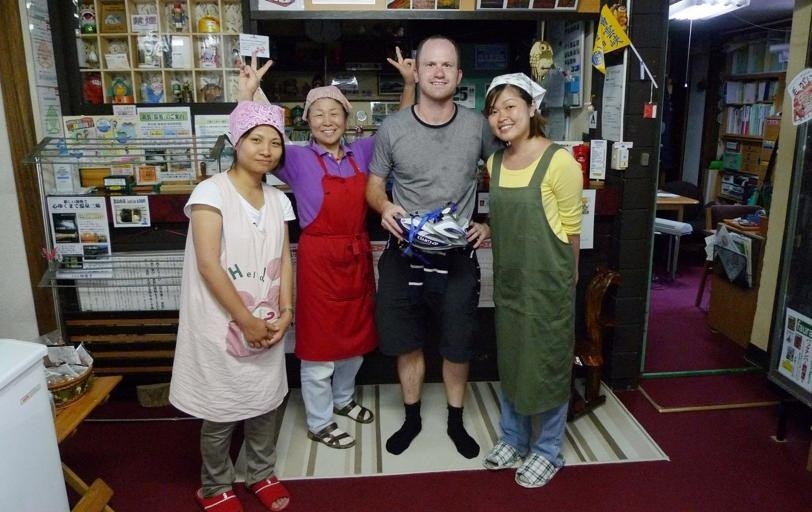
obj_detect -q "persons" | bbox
[168,100,297,511]
[365,34,507,460]
[481,72,583,490]
[227,47,419,448]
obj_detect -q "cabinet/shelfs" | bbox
[22,136,226,423]
[73,1,247,107]
[704,18,793,237]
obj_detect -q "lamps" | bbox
[668,0,752,21]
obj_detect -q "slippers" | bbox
[481,439,525,472]
[248,475,290,511]
[515,451,566,488]
[195,487,243,511]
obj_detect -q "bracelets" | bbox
[279,308,294,320]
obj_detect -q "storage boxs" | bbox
[742,145,760,173]
[764,118,780,141]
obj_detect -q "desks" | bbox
[53,376,127,512]
[656,190,699,282]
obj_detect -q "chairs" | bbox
[695,205,760,305]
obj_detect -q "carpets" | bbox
[231,377,672,489]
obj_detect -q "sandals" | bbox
[333,400,374,423]
[307,422,357,449]
